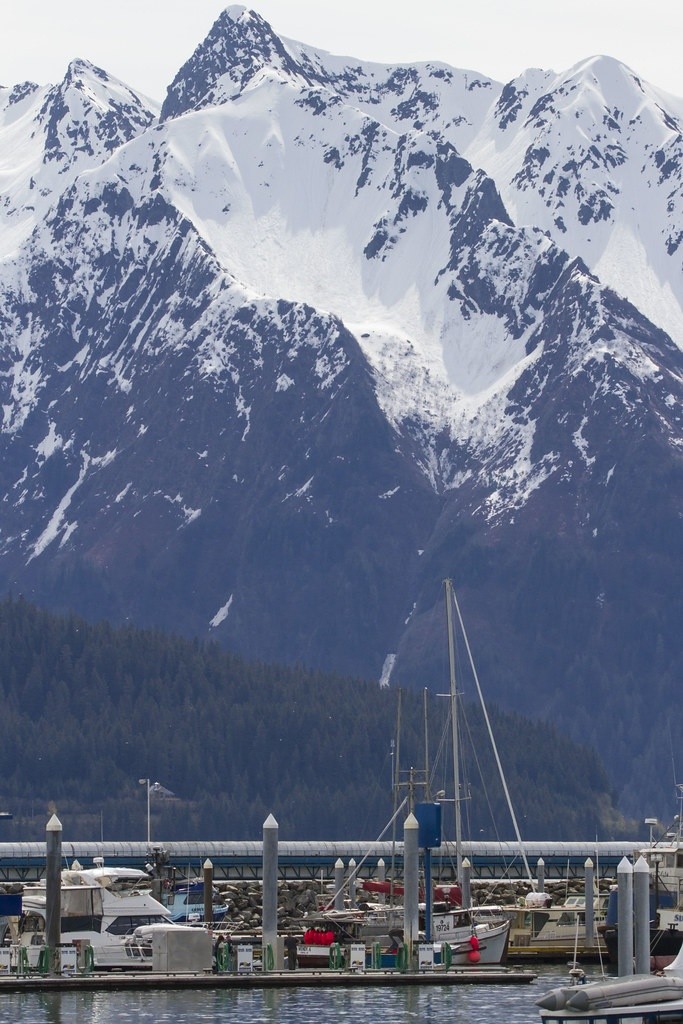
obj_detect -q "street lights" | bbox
[138,778,160,841]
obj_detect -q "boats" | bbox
[255,918,513,969]
[0,857,244,970]
[599,783,683,971]
[504,895,608,956]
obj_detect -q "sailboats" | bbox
[311,573,540,924]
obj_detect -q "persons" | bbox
[419,910,425,931]
[224,936,234,972]
[284,932,299,970]
[214,935,224,966]
